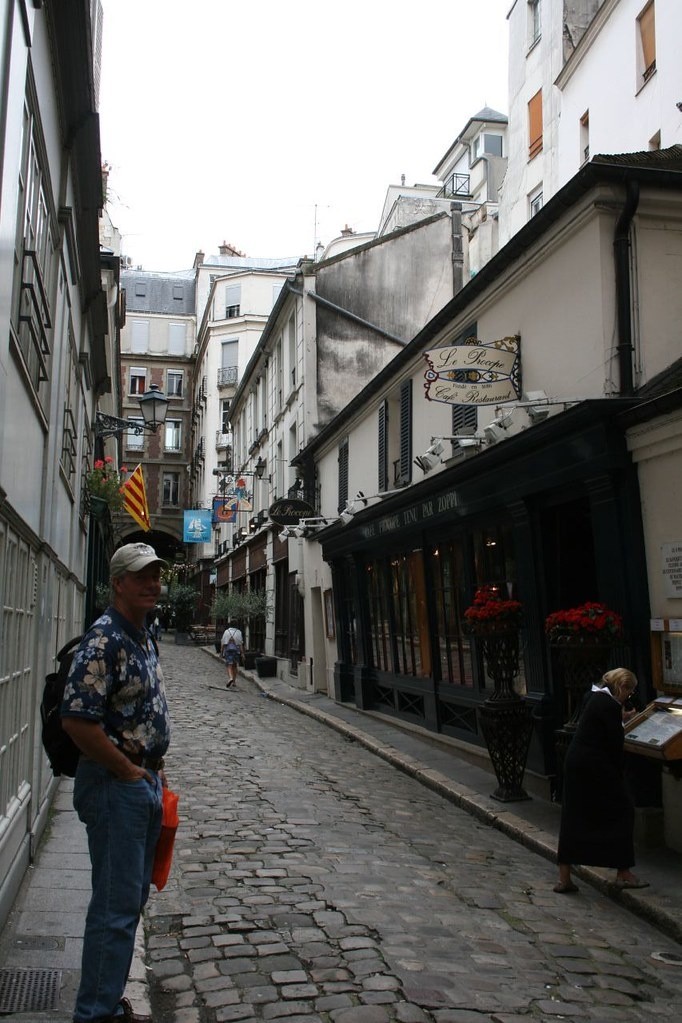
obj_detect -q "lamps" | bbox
[483,398,583,445]
[279,524,329,543]
[414,435,486,474]
[294,516,340,538]
[339,487,406,527]
[91,383,171,438]
[255,456,271,483]
[521,390,550,425]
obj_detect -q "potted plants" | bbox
[204,585,278,678]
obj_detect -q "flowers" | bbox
[465,584,523,635]
[84,456,127,511]
[546,603,622,644]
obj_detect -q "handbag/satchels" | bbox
[40,631,89,779]
[150,786,179,892]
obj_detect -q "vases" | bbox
[89,494,110,521]
[479,634,536,802]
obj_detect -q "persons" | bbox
[552,668,652,892]
[58,543,171,1023]
[162,605,171,633]
[146,608,162,641]
[221,620,245,688]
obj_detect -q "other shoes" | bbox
[226,678,235,688]
[616,876,650,889]
[120,997,152,1023]
[230,684,236,689]
[554,883,579,893]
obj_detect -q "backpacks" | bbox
[224,628,239,661]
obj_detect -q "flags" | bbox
[122,466,152,532]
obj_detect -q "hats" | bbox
[110,542,168,576]
